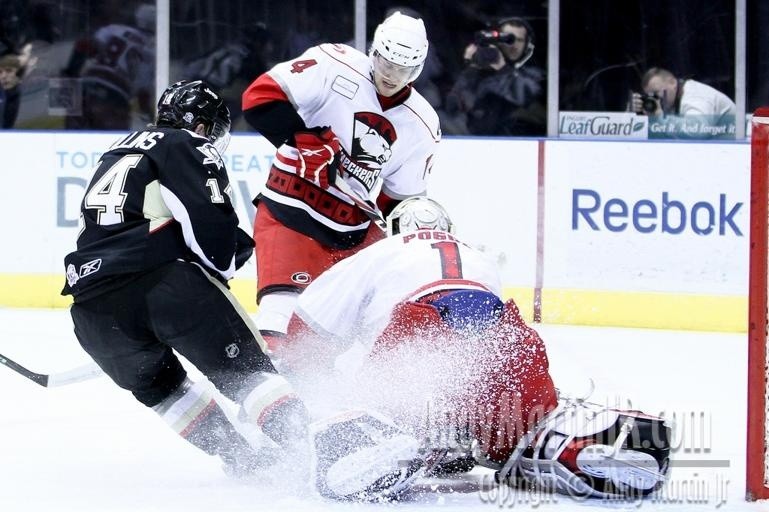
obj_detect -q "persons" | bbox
[0,0,737,139]
[281,193,672,504]
[240,9,445,366]
[61,78,312,486]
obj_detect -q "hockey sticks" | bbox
[0,355,106,387]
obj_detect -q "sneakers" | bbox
[326,434,420,497]
[566,439,661,491]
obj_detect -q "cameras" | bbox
[640,88,667,113]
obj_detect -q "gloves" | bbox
[293,125,345,190]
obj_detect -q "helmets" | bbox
[386,195,453,237]
[134,3,155,33]
[369,10,429,88]
[157,78,232,157]
